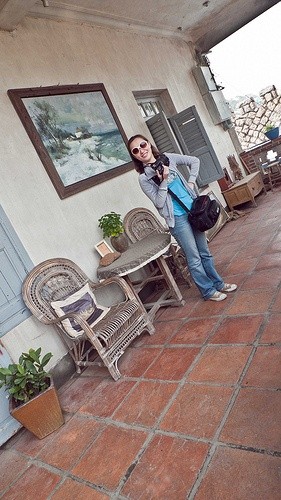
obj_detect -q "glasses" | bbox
[131,140,148,156]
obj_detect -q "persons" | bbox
[127,134,237,302]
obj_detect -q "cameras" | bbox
[151,155,170,175]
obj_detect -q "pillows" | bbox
[51,283,111,338]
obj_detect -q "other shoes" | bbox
[221,283,237,292]
[207,291,227,302]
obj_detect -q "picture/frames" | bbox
[96,240,114,256]
[7,83,136,200]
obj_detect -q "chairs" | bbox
[18,257,156,381]
[122,207,196,290]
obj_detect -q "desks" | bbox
[221,171,267,211]
[94,233,186,334]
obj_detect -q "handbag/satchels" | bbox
[188,194,220,232]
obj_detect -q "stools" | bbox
[266,161,281,191]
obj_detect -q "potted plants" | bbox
[97,212,130,253]
[0,348,65,440]
[264,125,279,140]
